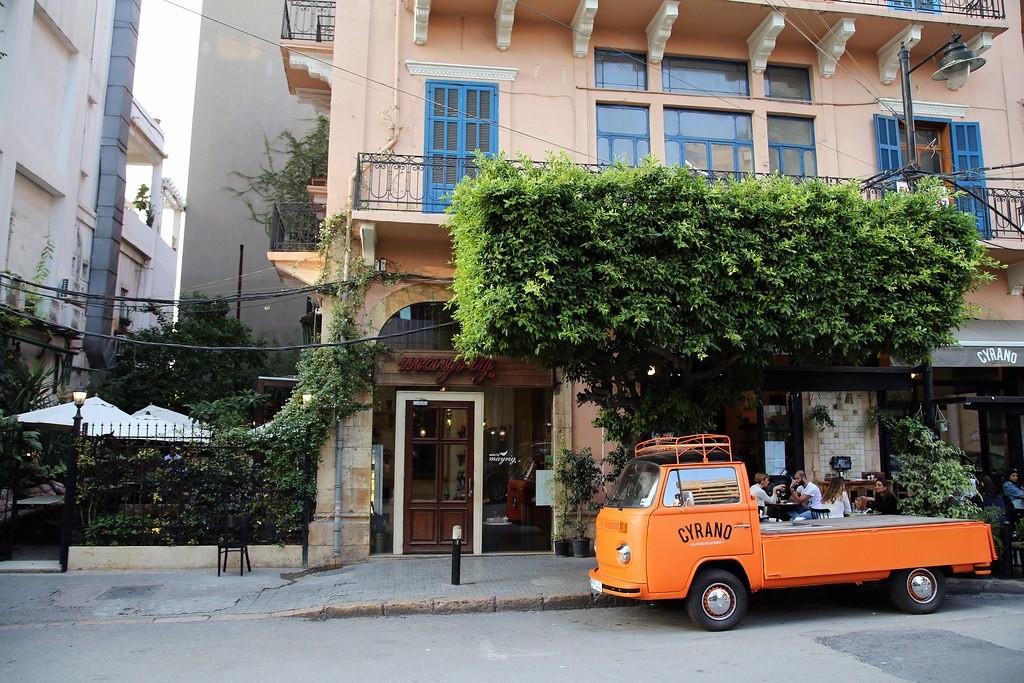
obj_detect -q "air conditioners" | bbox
[59,277,75,299]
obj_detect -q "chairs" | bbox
[218,516,251,576]
[758,506,769,523]
[1003,495,1024,533]
[810,506,830,519]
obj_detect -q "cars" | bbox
[889,445,1017,577]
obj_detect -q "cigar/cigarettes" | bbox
[790,476,793,480]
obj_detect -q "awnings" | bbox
[891,318,1024,368]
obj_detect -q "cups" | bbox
[487,515,509,523]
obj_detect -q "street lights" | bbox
[59,384,88,573]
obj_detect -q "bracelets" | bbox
[791,488,795,492]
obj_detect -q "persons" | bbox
[965,468,1024,526]
[857,477,900,516]
[750,473,786,522]
[163,445,184,464]
[788,470,822,521]
[821,476,852,518]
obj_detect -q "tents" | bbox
[3,391,218,443]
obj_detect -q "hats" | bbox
[1006,467,1018,479]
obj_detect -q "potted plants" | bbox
[806,405,837,432]
[545,434,605,558]
[933,419,950,432]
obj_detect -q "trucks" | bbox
[458,441,552,533]
[587,433,999,632]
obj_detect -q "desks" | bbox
[817,481,876,502]
[844,513,882,517]
[765,502,801,522]
[16,495,63,522]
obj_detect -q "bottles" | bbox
[776,488,787,503]
